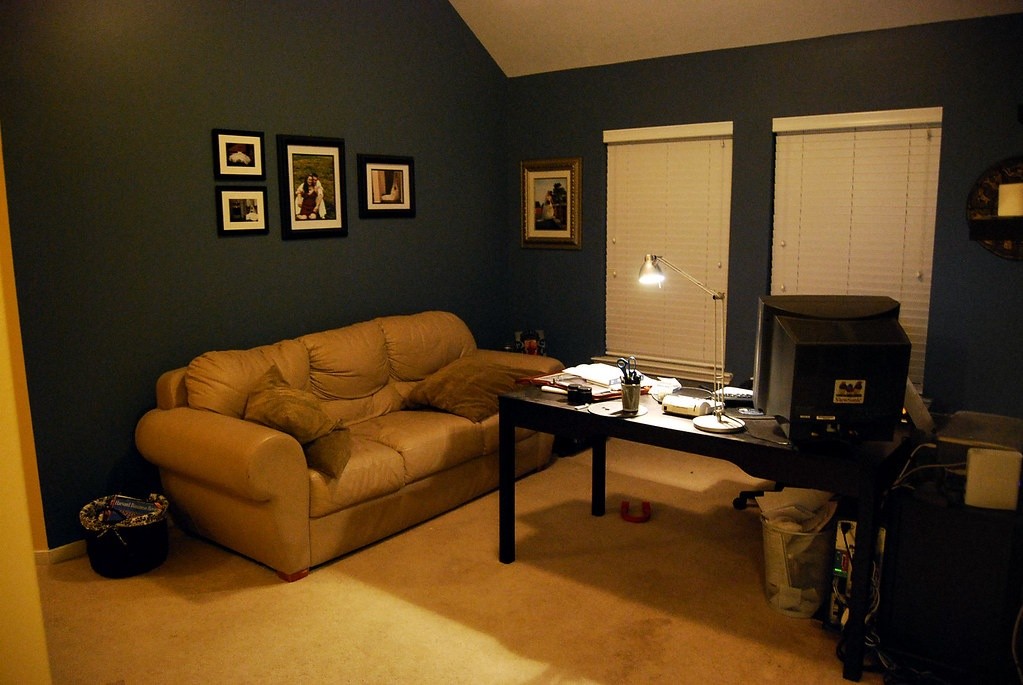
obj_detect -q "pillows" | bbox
[400,355,530,423]
[242,363,352,479]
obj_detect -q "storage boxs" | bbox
[80,493,168,580]
[757,510,829,619]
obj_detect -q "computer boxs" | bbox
[821,483,903,635]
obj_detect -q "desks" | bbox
[497,358,928,682]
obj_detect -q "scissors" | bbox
[617,356,636,385]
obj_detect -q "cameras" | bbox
[567,384,592,404]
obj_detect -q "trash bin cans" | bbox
[760,516,832,619]
[79,493,171,579]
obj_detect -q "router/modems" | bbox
[964,448,1023,510]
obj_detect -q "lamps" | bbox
[636,254,746,434]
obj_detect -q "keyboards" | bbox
[711,393,753,406]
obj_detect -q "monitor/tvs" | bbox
[752,295,912,446]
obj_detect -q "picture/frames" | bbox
[275,133,350,242]
[210,128,267,182]
[215,185,270,239]
[356,153,417,220]
[521,155,583,250]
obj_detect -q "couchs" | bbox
[136,309,568,584]
